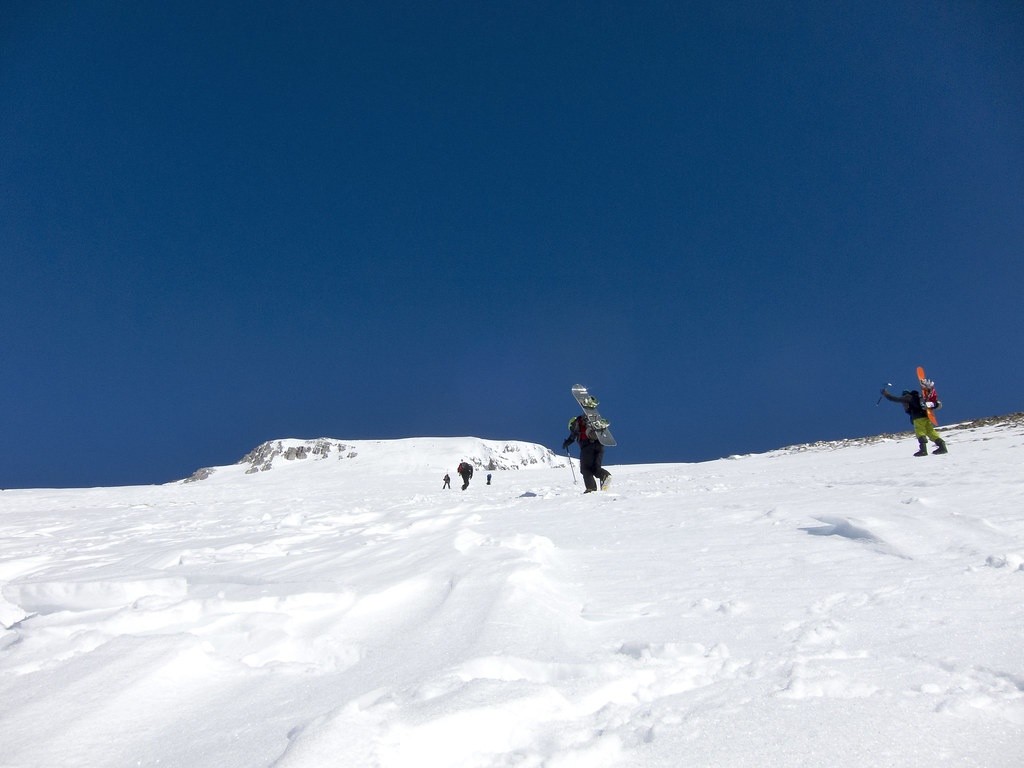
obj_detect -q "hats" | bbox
[902,391,910,396]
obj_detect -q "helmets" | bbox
[568,417,577,431]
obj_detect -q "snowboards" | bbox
[916,366,942,427]
[571,383,618,448]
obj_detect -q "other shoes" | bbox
[583,487,597,494]
[601,470,612,492]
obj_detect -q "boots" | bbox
[933,442,947,454]
[914,444,927,456]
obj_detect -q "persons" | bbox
[562,415,613,494]
[457,462,473,491]
[880,387,949,458]
[442,474,451,489]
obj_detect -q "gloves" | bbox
[563,438,574,449]
[881,389,890,397]
